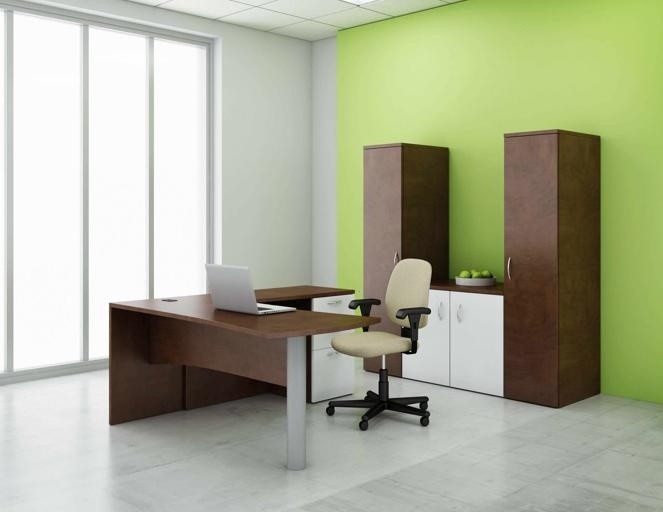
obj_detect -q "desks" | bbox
[108,286,356,471]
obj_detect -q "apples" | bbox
[459,268,492,278]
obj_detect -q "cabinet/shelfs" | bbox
[401,281,504,397]
[504,129,600,407]
[363,144,447,377]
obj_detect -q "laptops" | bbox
[205,263,297,315]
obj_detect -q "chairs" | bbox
[326,258,433,431]
